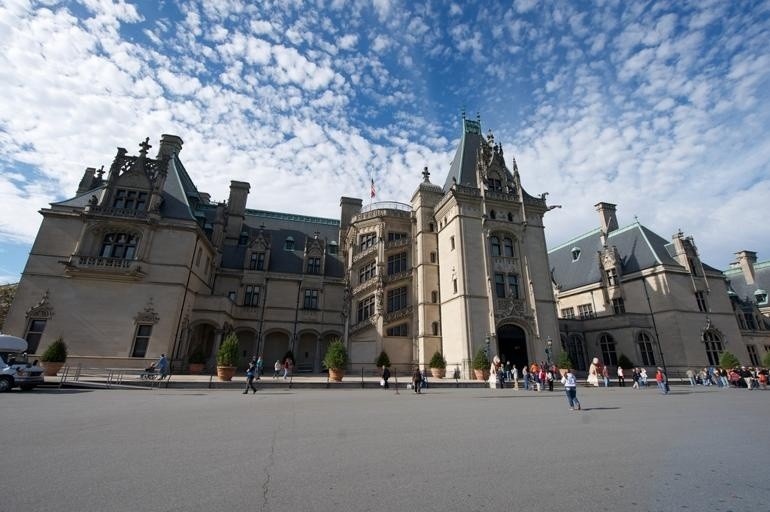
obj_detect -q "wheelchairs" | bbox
[142,366,158,380]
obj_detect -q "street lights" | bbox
[546,334,555,363]
[485,334,492,367]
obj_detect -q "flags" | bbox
[370,178,376,199]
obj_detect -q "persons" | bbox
[560,368,581,410]
[602,364,610,386]
[153,351,171,387]
[412,367,428,395]
[494,359,560,392]
[256,355,293,384]
[631,365,671,394]
[617,365,625,386]
[240,360,258,395]
[138,362,155,380]
[381,364,391,389]
[686,365,770,391]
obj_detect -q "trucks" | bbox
[0,334,45,392]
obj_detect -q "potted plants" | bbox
[469,346,494,382]
[214,331,240,381]
[322,335,351,383]
[429,350,447,378]
[373,350,391,375]
[38,336,71,378]
[556,348,576,381]
